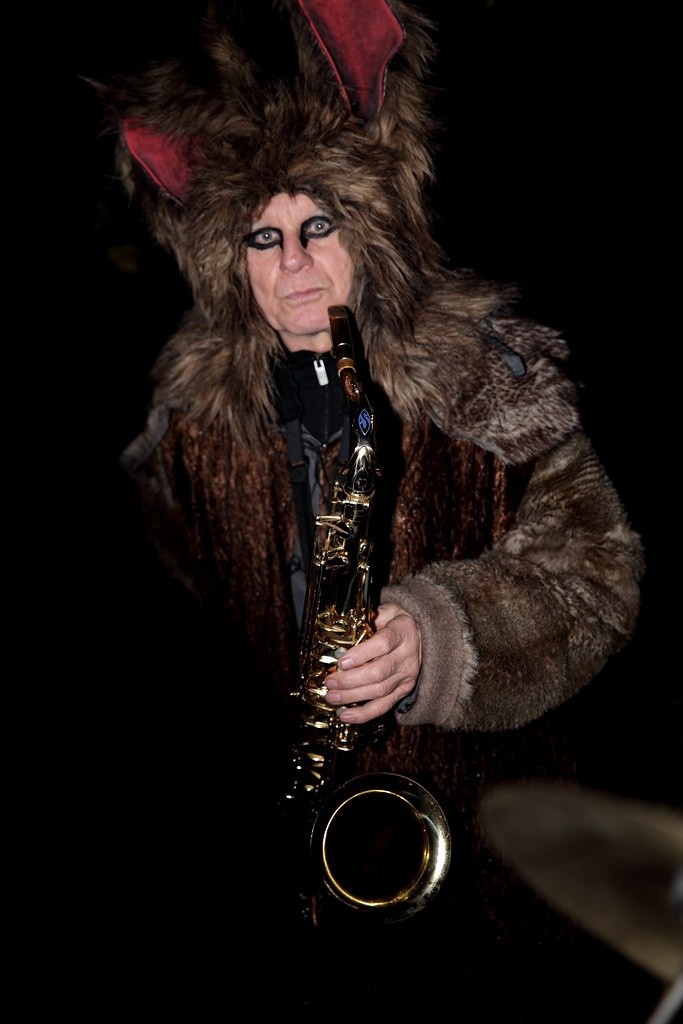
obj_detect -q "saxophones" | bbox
[272,305,450,941]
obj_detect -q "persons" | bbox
[78,1,647,1024]
